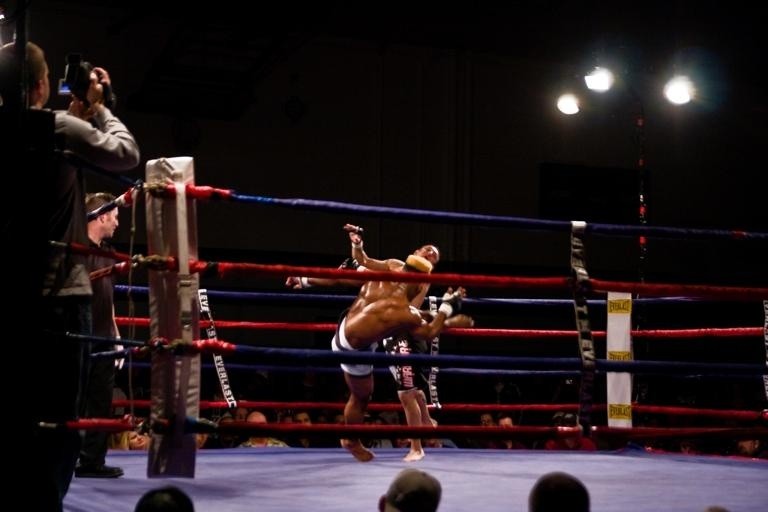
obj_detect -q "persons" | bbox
[545,411,597,451]
[378,469,442,511]
[474,413,525,450]
[276,221,473,462]
[104,391,413,451]
[738,439,768,458]
[528,471,590,511]
[77,188,134,479]
[136,485,194,512]
[0,41,141,512]
[337,223,441,462]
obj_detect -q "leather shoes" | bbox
[75,464,123,477]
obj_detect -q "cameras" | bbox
[57,58,90,98]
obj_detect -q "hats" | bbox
[407,255,432,273]
[386,468,440,511]
